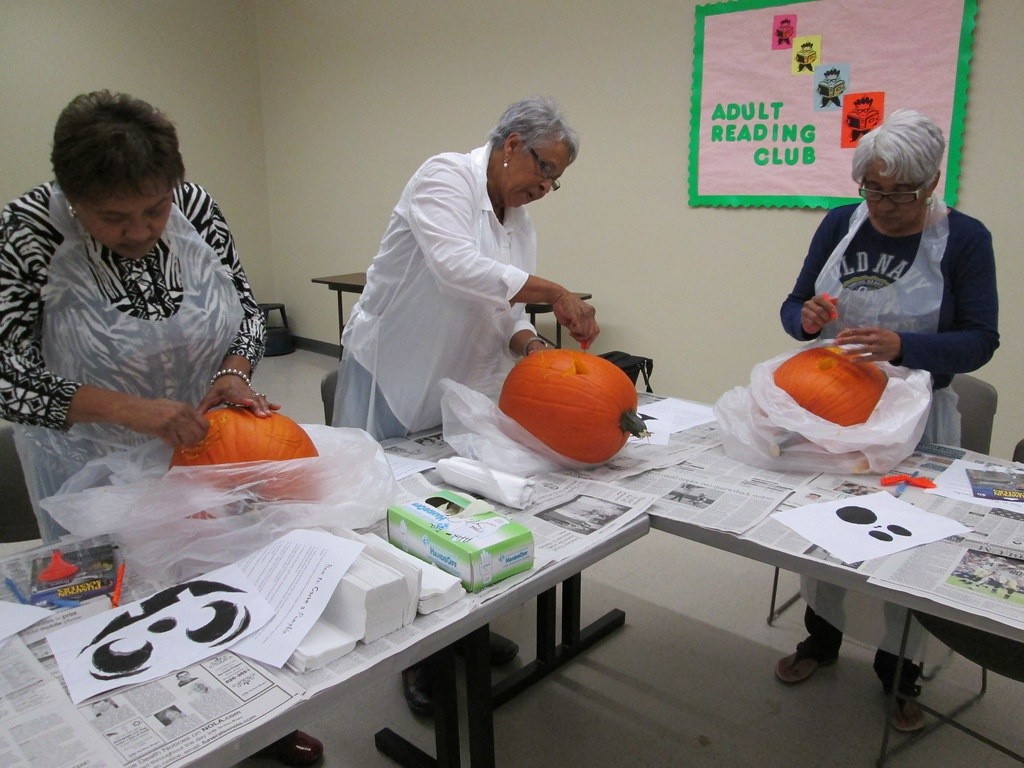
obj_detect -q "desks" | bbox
[405,392,1024,709]
[0,391,652,768]
[311,272,593,366]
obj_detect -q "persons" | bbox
[835,483,877,496]
[806,493,819,501]
[0,91,324,768]
[92,699,118,718]
[158,709,185,724]
[672,484,707,507]
[193,682,212,694]
[1013,537,1024,544]
[541,481,558,491]
[177,669,198,688]
[777,110,1000,731]
[331,99,600,717]
[951,553,1024,599]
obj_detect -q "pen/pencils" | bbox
[4,577,27,603]
[893,470,919,498]
[113,558,125,607]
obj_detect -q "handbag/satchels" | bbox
[438,373,626,477]
[714,339,935,475]
[37,422,398,592]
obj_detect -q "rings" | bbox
[255,393,266,399]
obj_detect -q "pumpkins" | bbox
[167,406,320,518]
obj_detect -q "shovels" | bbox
[822,292,859,335]
[581,342,587,352]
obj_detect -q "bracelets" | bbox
[211,369,251,407]
[524,338,547,357]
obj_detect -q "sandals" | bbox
[883,683,926,732]
[776,642,839,684]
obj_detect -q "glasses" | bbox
[858,176,931,204]
[522,140,561,191]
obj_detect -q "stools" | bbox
[256,302,297,357]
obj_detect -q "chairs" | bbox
[766,372,998,631]
[876,439,1024,768]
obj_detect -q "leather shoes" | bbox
[253,730,323,766]
[401,663,438,713]
[455,631,518,666]
